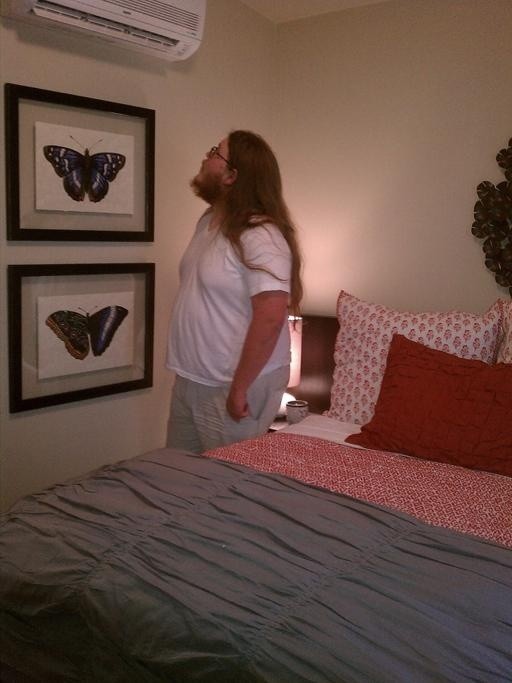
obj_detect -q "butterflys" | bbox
[41,133,126,203]
[44,303,129,361]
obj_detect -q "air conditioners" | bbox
[0,0,206,62]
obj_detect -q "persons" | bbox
[163,128,302,452]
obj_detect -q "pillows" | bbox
[324,289,511,481]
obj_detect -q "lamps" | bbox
[0,308,512,681]
[277,312,304,419]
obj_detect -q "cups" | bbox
[285,400,309,422]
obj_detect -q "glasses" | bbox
[208,145,233,168]
